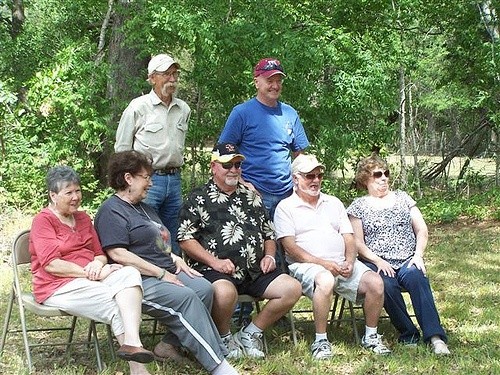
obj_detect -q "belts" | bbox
[153,167,180,176]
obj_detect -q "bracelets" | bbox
[266,255,276,262]
[157,269,167,280]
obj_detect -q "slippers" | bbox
[151,346,192,362]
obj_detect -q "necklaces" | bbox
[115,194,162,228]
[54,208,75,233]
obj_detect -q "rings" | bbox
[109,264,113,267]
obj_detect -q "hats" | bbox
[254,57,286,79]
[210,142,245,163]
[291,153,325,175]
[148,54,180,74]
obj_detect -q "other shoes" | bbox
[432,340,450,356]
[220,331,243,358]
[361,332,391,355]
[310,339,334,360]
[233,325,265,360]
[116,344,154,364]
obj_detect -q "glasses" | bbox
[217,161,241,169]
[299,172,325,180]
[369,170,389,178]
[136,174,150,180]
[153,70,180,78]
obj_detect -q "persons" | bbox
[28,166,154,375]
[217,57,309,327]
[347,155,451,355]
[176,144,302,359]
[115,54,191,260]
[273,154,391,360]
[94,151,240,375]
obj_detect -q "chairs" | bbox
[0,229,103,373]
[87,239,408,363]
